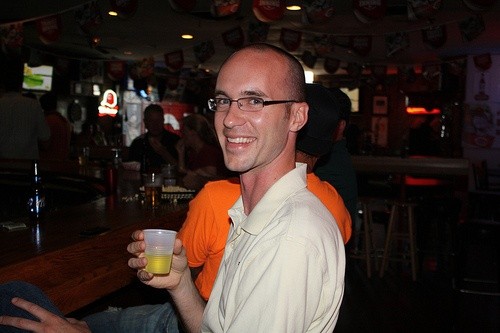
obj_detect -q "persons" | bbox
[402,115,454,273]
[1,42,352,333]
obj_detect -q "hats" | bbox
[296,85,341,156]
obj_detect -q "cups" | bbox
[144,179,162,209]
[142,229,176,274]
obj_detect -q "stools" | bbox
[348,199,421,280]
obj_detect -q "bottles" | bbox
[103,167,118,210]
[27,163,46,219]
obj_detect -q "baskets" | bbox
[156,186,198,203]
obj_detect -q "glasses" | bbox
[208,97,296,112]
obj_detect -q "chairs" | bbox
[446,190,500,296]
